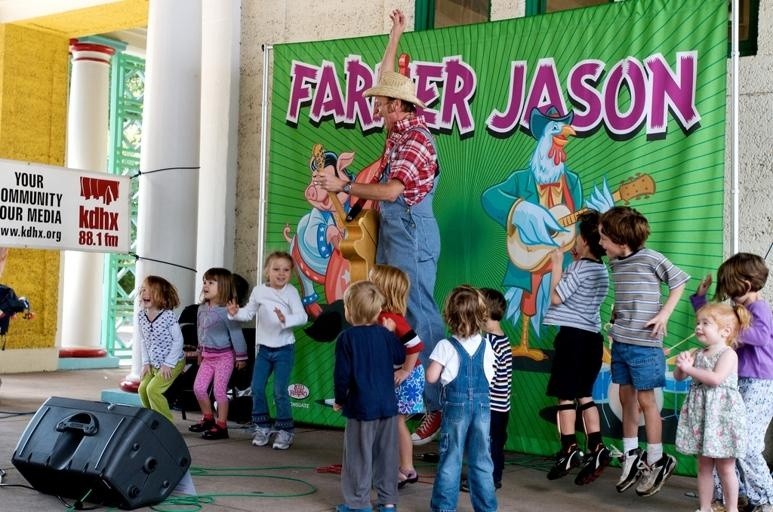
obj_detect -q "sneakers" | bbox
[575,443,613,486]
[609,443,676,497]
[252,423,274,446]
[377,502,397,512]
[336,503,373,512]
[547,443,587,480]
[684,487,772,512]
[397,466,419,488]
[461,478,470,492]
[411,409,443,446]
[273,429,294,450]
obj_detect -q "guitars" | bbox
[506,173,656,273]
[313,143,378,285]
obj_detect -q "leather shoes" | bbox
[201,424,229,440]
[188,418,216,432]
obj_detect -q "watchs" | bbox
[344,182,352,194]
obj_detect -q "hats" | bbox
[361,69,428,109]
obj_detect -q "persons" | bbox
[189,268,249,440]
[542,210,613,486]
[597,205,691,498]
[425,284,497,512]
[690,252,773,512]
[674,304,753,512]
[226,251,308,450]
[318,9,447,445]
[333,281,406,512]
[370,265,425,489]
[138,276,186,422]
[460,287,512,493]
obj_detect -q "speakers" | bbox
[11,393,191,510]
